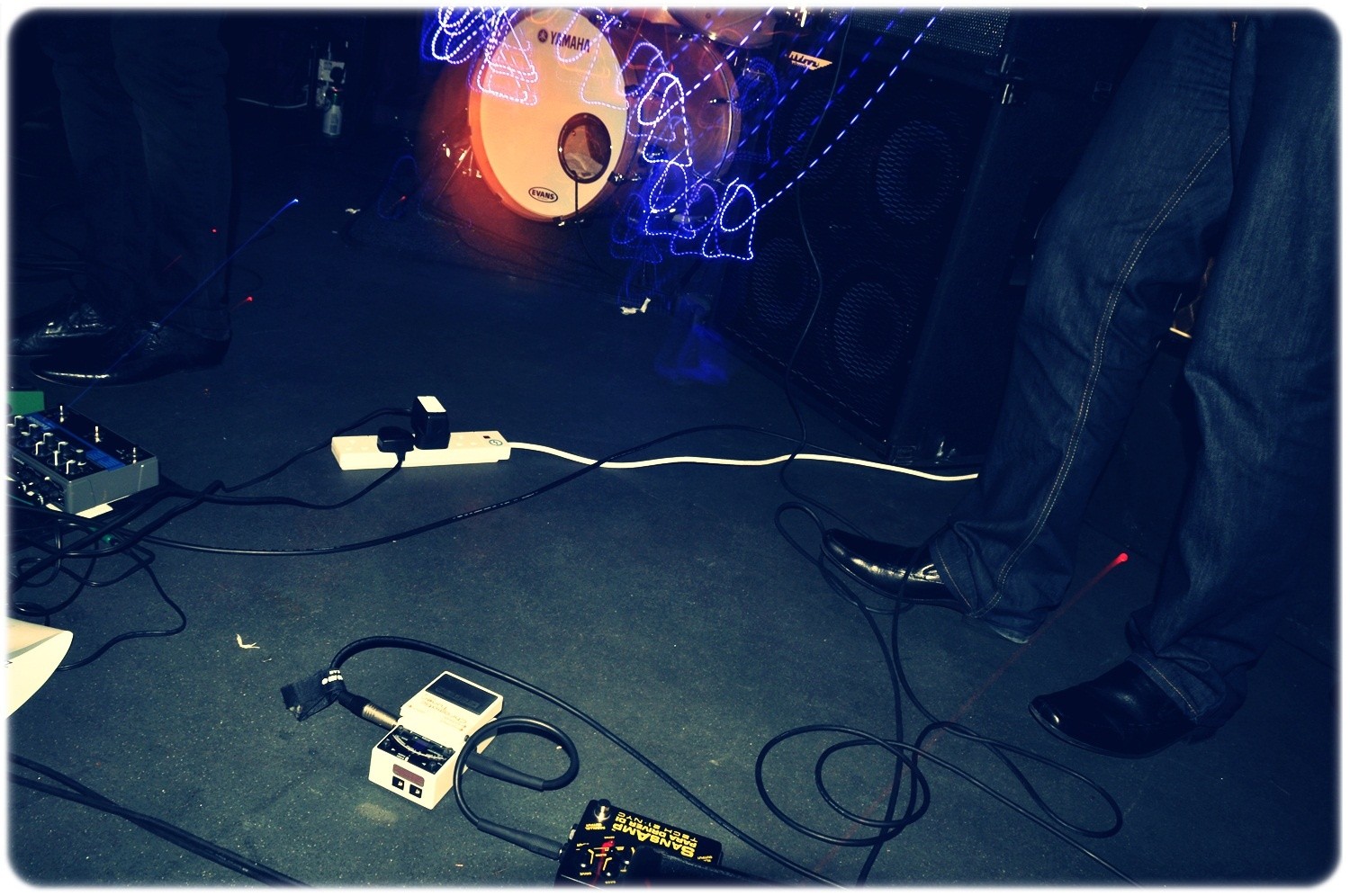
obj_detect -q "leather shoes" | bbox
[13,293,122,355]
[1027,660,1236,757]
[30,339,232,387]
[818,530,1037,647]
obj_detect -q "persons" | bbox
[830,6,1343,755]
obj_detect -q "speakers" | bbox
[705,10,1154,466]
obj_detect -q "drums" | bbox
[465,9,743,227]
[667,8,775,51]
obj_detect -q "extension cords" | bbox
[332,431,512,469]
